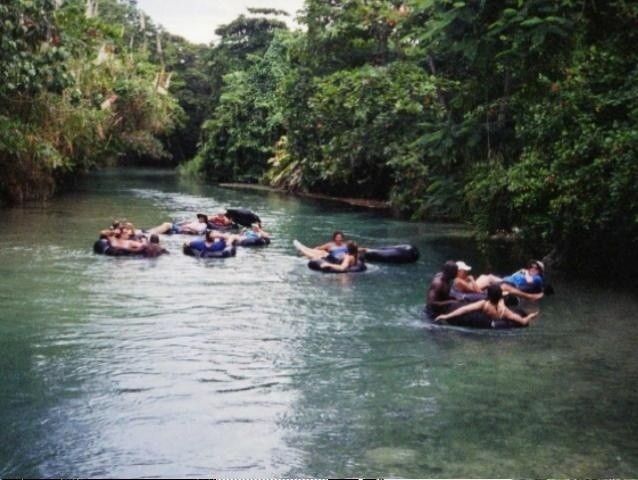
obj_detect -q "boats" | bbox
[423,271,529,330]
[307,242,421,273]
[93,207,271,258]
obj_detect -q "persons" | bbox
[293,239,358,271]
[302,231,367,260]
[99,206,276,257]
[432,259,544,302]
[433,285,539,325]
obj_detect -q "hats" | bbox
[531,260,545,276]
[456,260,471,271]
